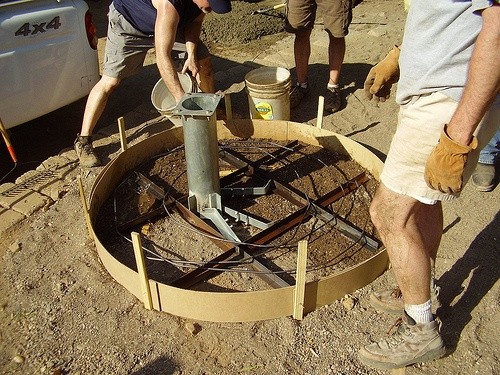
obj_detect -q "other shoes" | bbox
[471,161,496,192]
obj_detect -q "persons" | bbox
[356,0,500,371]
[285,0,363,113]
[72,0,231,168]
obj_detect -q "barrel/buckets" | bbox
[245,48,291,123]
[151,71,198,126]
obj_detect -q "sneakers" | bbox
[357,310,447,369]
[289,83,309,107]
[324,82,342,112]
[370,286,445,315]
[74,133,101,167]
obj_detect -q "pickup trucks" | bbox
[0,0,102,134]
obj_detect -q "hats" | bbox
[208,0,232,14]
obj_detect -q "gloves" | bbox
[364,44,402,102]
[423,124,478,197]
